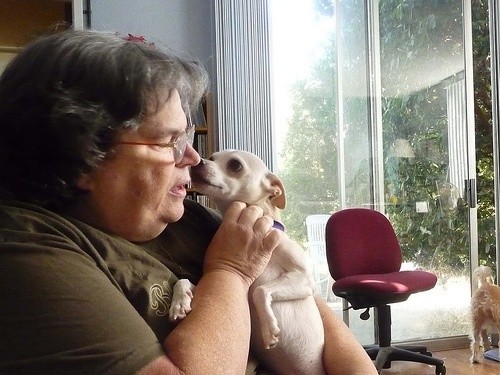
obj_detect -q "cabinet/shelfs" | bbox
[181,93,214,211]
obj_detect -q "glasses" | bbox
[115,124,196,163]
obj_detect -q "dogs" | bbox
[468,266,500,364]
[167,149,323,375]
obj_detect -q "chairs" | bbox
[326,208,447,374]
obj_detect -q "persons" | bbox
[0,30,381,375]
